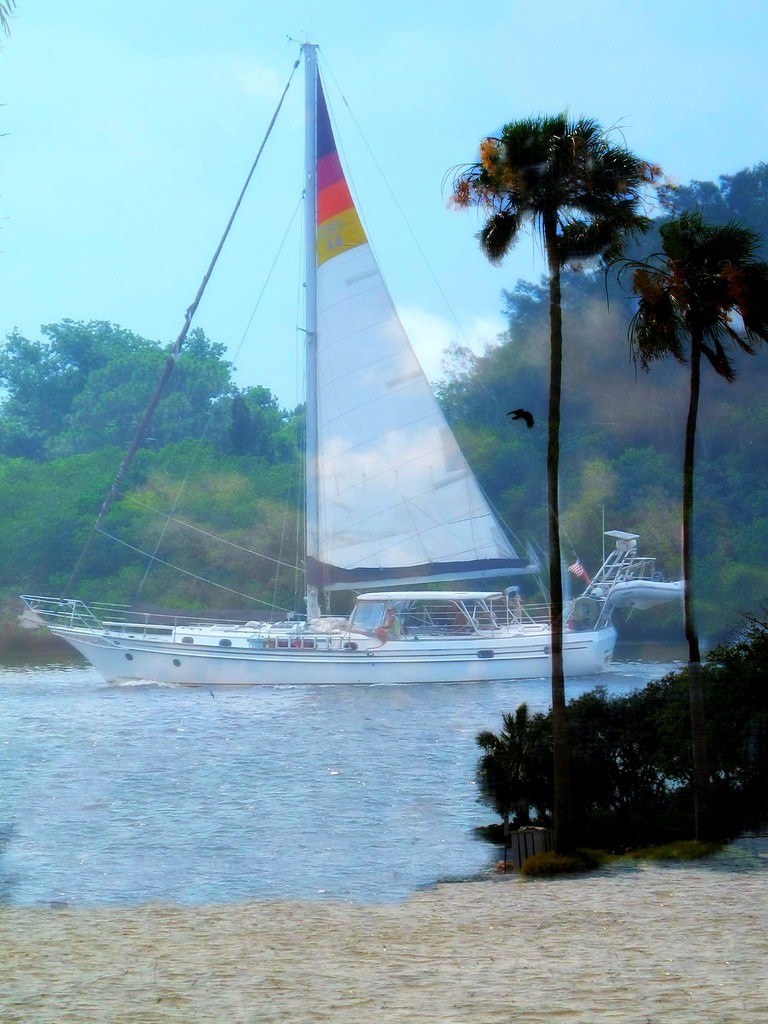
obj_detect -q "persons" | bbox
[375,606,402,643]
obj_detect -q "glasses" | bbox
[387,609,392,611]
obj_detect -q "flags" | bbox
[567,556,586,578]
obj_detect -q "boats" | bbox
[609,557,684,611]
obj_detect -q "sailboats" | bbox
[15,30,643,685]
